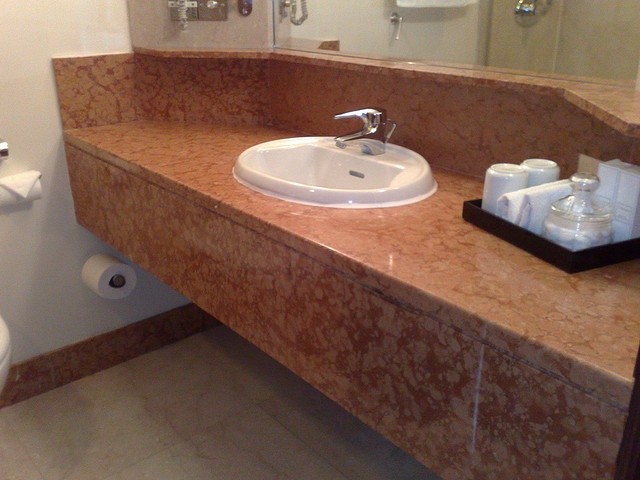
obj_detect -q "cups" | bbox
[482,162,526,216]
[520,159,560,188]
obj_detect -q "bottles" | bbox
[543,174,615,252]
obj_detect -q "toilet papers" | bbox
[81,252,138,301]
[0,170,43,208]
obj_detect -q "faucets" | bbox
[334,107,388,153]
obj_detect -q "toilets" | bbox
[0,315,10,397]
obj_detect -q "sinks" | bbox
[234,136,431,202]
